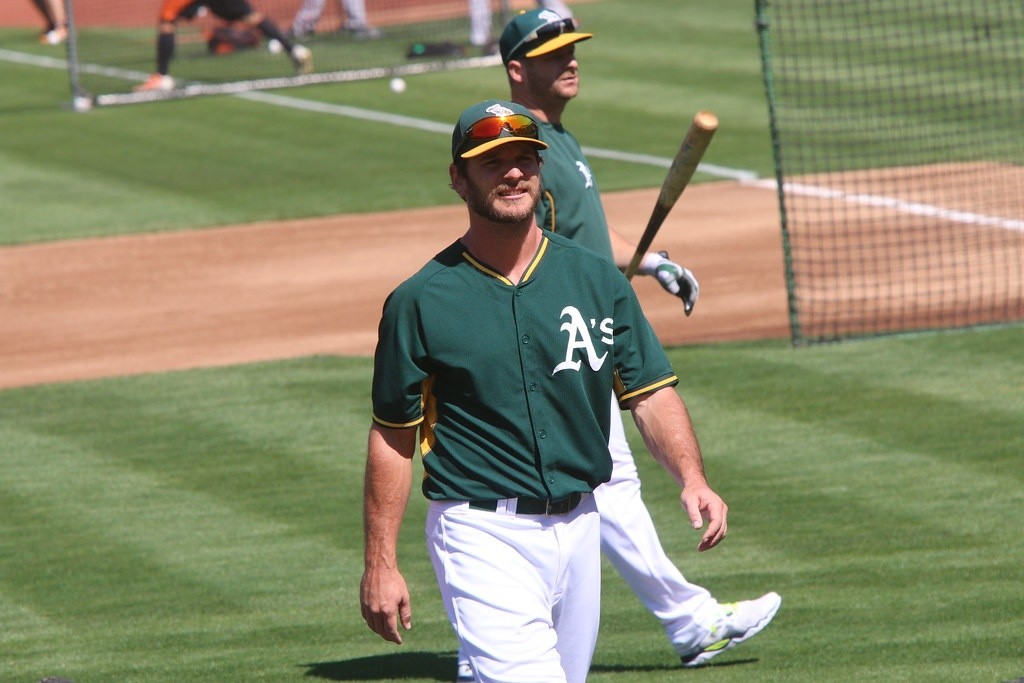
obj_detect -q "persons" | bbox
[498,5,782,669]
[357,100,729,683]
[130,0,381,90]
[468,0,579,47]
[34,0,68,47]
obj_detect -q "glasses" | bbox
[513,17,575,58]
[453,114,539,162]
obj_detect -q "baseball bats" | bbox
[621,109,720,282]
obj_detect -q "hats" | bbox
[452,99,549,163]
[499,9,593,64]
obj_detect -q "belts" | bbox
[469,492,582,514]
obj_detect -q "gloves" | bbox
[644,251,700,317]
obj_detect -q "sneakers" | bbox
[681,592,781,667]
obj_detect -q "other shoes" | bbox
[291,43,314,75]
[344,26,382,40]
[39,25,69,46]
[292,19,309,44]
[130,73,176,93]
